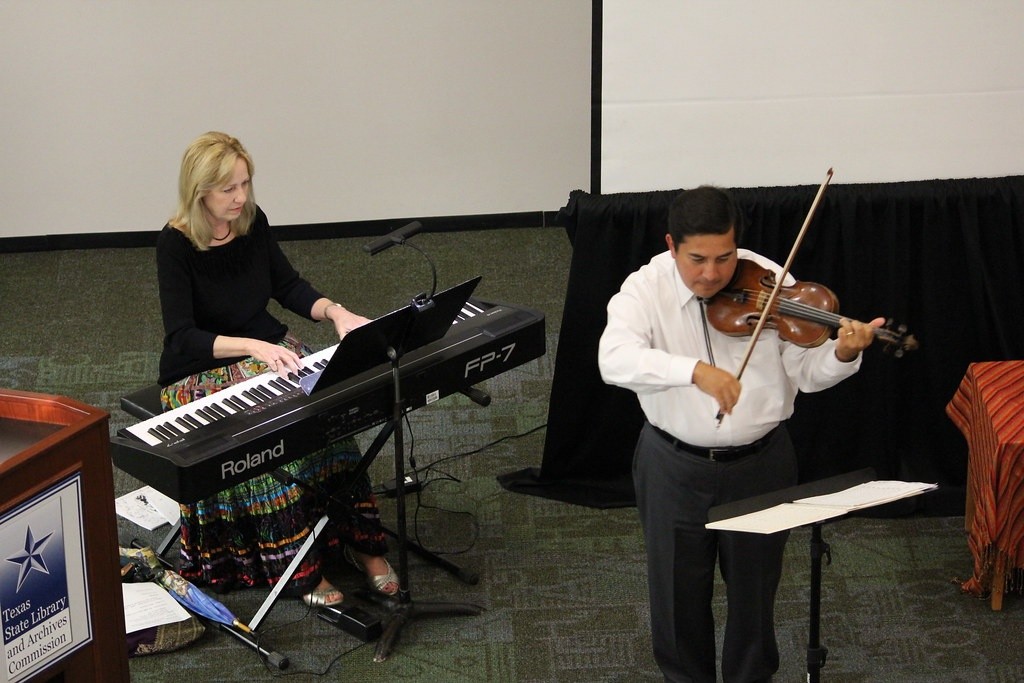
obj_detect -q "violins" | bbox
[705,256,921,362]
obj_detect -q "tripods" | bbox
[298,273,487,662]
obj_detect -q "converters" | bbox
[381,475,420,499]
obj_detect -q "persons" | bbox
[155,130,400,608]
[596,185,886,683]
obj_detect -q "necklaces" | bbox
[213,221,231,241]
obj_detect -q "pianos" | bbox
[110,291,547,510]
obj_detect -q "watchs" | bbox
[323,303,341,321]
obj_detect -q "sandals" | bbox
[303,584,344,608]
[346,548,401,596]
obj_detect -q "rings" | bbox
[846,331,853,336]
[275,358,281,362]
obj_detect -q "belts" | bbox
[654,422,782,463]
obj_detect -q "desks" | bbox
[945,360,1024,610]
[1,388,132,683]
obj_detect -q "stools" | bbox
[122,385,168,420]
